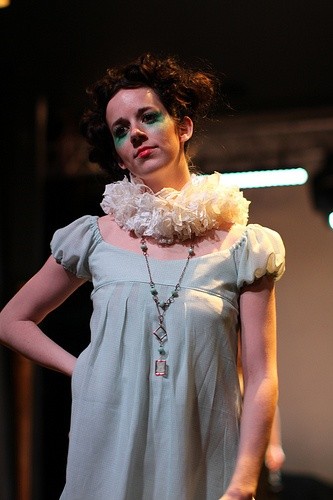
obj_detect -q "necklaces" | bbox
[136,233,196,376]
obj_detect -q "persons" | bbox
[0,53,287,500]
[230,326,286,500]
